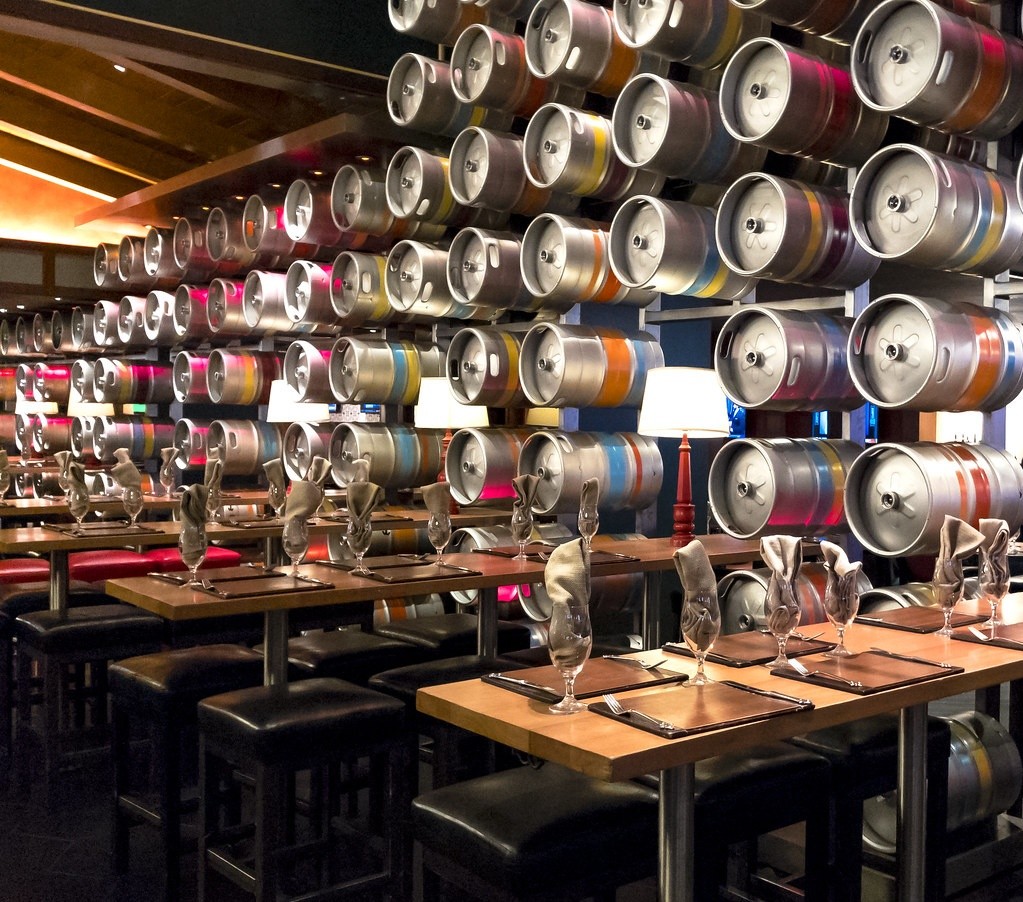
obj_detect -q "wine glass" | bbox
[347,518,372,574]
[548,605,593,713]
[282,522,309,577]
[427,514,451,565]
[160,469,174,494]
[509,509,533,559]
[932,558,964,636]
[578,510,599,552]
[681,593,722,686]
[0,471,10,503]
[178,528,207,588]
[824,573,860,657]
[124,489,143,528]
[57,474,70,501]
[68,489,89,532]
[206,490,221,524]
[764,578,802,669]
[978,555,1011,627]
[268,487,286,519]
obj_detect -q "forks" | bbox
[969,627,1023,646]
[538,551,549,560]
[788,658,863,686]
[398,553,430,560]
[603,654,668,669]
[202,579,229,595]
[603,694,673,729]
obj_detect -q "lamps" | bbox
[14,378,59,458]
[414,377,489,515]
[66,382,116,470]
[266,379,330,494]
[637,367,731,548]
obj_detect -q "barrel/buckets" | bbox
[0,0,1023,556]
[863,711,1023,853]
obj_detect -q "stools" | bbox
[0,544,955,902]
[0,533,1023,902]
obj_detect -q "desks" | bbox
[416,591,1023,902]
[0,505,513,781]
[0,488,423,559]
[105,533,843,896]
